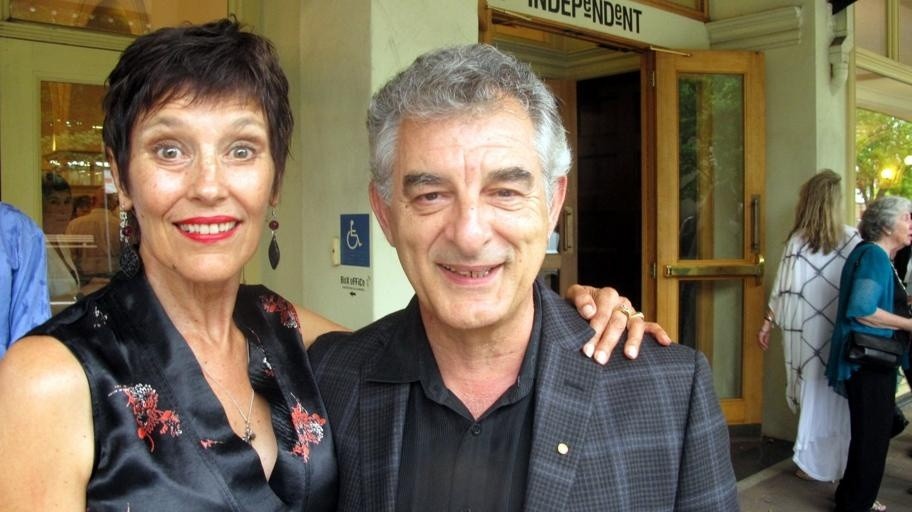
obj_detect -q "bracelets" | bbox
[763,316,772,323]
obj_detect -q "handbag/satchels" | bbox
[890,402,908,439]
[843,331,903,368]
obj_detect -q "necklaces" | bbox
[871,241,909,296]
[190,340,258,446]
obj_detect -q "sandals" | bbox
[871,500,887,512]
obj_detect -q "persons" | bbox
[758,167,861,482]
[831,194,912,510]
[306,44,741,511]
[0,191,52,363]
[0,14,673,512]
[895,243,912,388]
[44,168,125,313]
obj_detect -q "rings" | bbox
[630,312,645,320]
[615,305,631,320]
